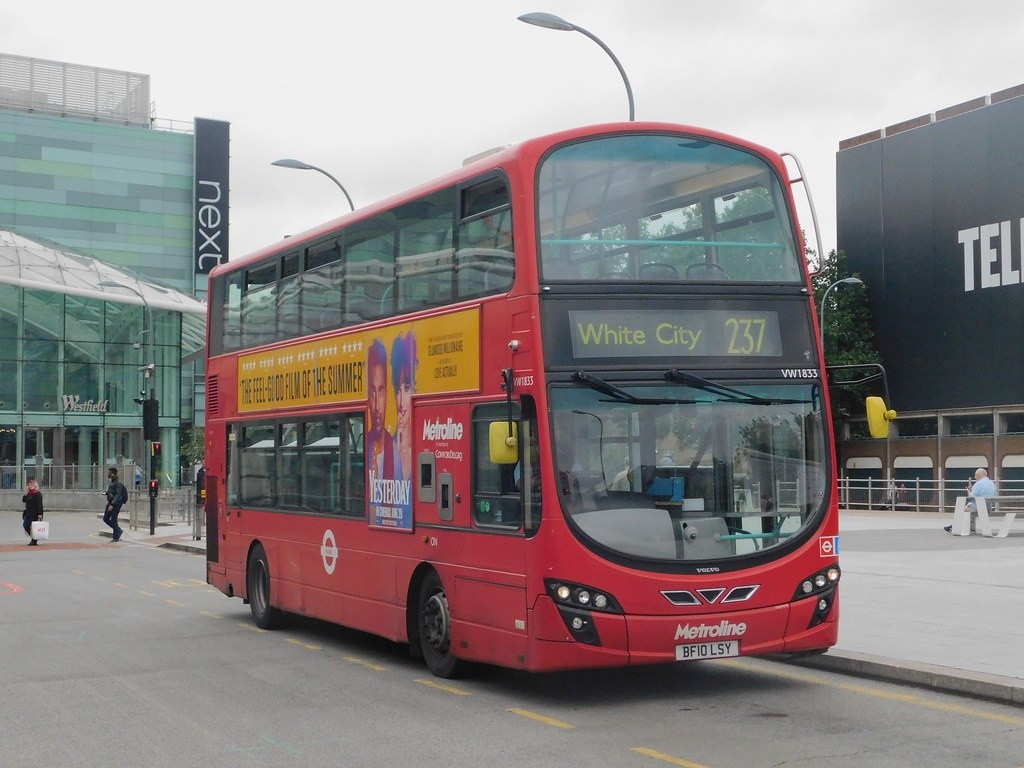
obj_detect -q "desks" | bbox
[952,496,1024,536]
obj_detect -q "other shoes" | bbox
[944,525,952,532]
[111,540,118,542]
[119,530,123,538]
[28,539,37,546]
[970,529,976,535]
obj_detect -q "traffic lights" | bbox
[149,479,158,500]
[154,442,160,456]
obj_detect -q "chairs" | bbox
[226,269,491,350]
[637,262,683,281]
[542,258,581,279]
[685,263,733,281]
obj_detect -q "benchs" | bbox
[960,510,1024,538]
[227,447,367,518]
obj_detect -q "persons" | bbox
[2,459,13,489]
[888,483,907,510]
[368,332,419,529]
[514,412,596,497]
[660,449,676,466]
[944,468,995,531]
[743,472,758,509]
[102,468,123,542]
[135,464,143,491]
[22,478,43,546]
[612,456,631,492]
[194,457,206,540]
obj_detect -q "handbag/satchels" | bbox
[23,510,26,519]
[30,518,49,540]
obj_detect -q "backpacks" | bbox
[119,482,128,504]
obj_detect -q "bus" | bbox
[203,122,898,680]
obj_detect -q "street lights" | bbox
[269,159,361,215]
[818,277,863,355]
[98,281,153,405]
[516,8,636,123]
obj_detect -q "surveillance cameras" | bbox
[133,343,141,350]
[138,366,148,372]
[147,364,155,371]
